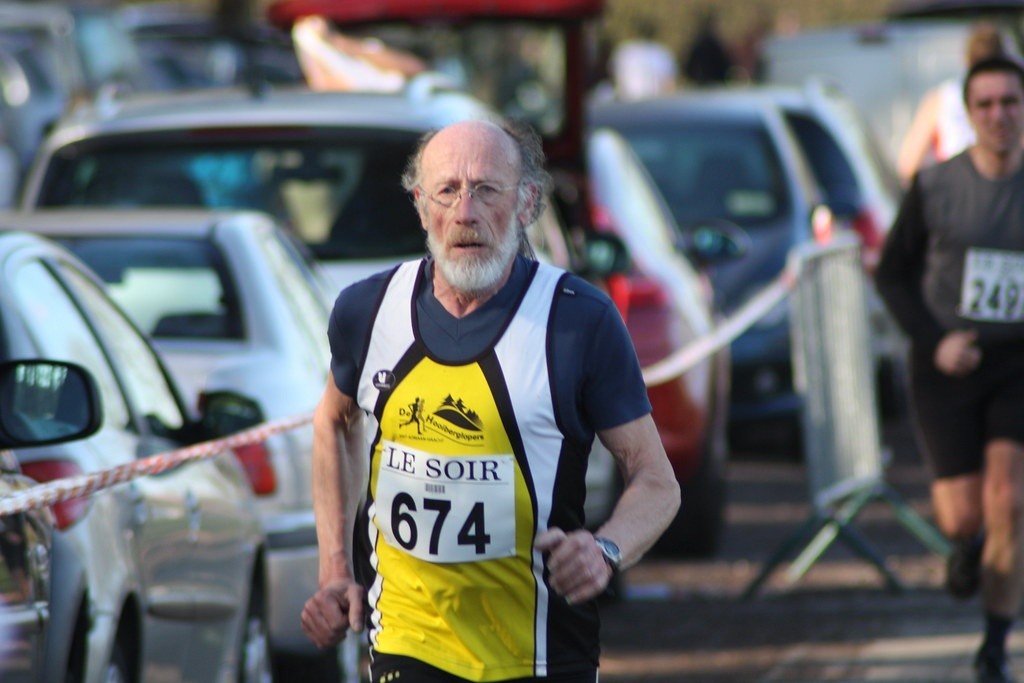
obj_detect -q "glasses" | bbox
[418,183,520,206]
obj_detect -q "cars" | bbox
[0,0,1024,683]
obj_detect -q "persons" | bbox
[302,118,684,683]
[894,22,1010,190]
[611,5,785,107]
[869,52,1023,683]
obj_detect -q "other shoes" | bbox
[947,532,983,597]
[974,615,1011,683]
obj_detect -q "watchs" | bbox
[592,533,622,576]
